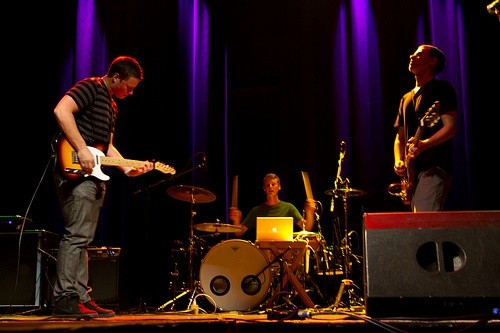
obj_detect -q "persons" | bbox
[392,45,459,212]
[227,174,318,239]
[46,53,155,318]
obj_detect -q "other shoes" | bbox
[52,293,114,319]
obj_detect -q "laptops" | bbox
[256,217,294,242]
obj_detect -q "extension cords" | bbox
[181,309,206,314]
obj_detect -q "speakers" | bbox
[361,210,500,317]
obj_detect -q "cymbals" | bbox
[325,188,363,196]
[166,185,217,203]
[193,223,244,233]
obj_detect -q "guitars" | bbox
[401,99,441,205]
[58,137,176,182]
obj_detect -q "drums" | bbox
[199,239,272,312]
